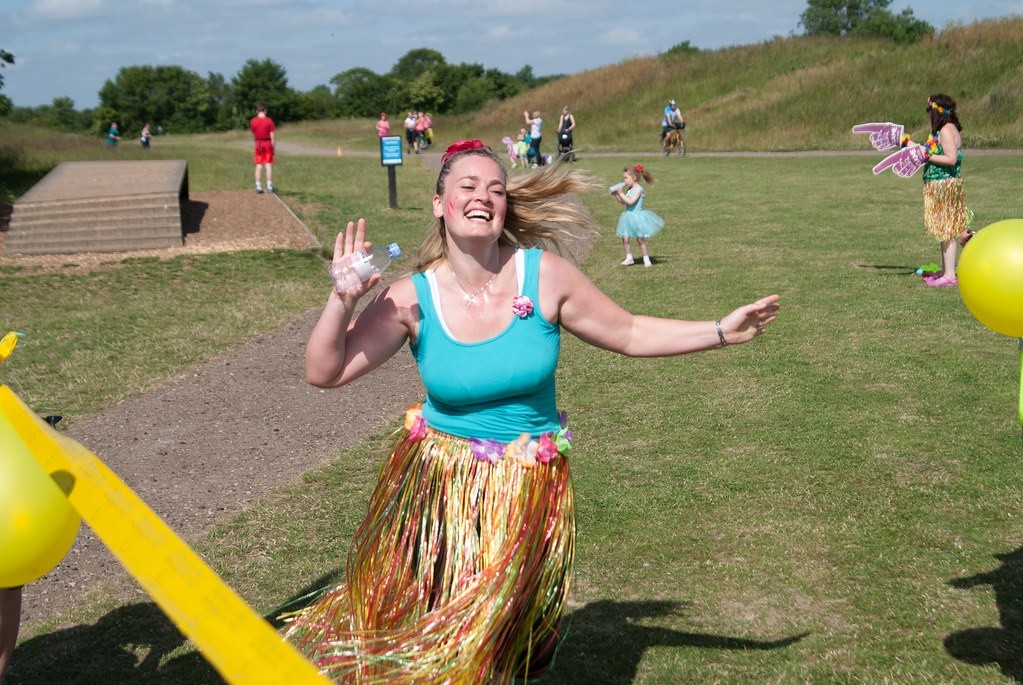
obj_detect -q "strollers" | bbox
[555,128,576,163]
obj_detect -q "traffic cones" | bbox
[337,145,344,156]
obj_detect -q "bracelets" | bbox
[716,320,729,347]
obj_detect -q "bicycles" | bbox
[658,121,688,158]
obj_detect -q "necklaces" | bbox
[445,252,502,308]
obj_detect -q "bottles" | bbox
[609,183,626,194]
[327,243,402,294]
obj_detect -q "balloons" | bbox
[957,217,1023,343]
[1,410,83,587]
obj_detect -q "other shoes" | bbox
[645,261,652,267]
[621,258,635,266]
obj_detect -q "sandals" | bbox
[924,273,958,288]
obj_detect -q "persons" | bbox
[959,228,1023,429]
[852,92,975,288]
[140,124,152,149]
[609,164,666,268]
[104,123,121,148]
[661,98,684,148]
[403,111,432,154]
[502,109,544,170]
[555,105,576,163]
[250,105,277,194]
[377,112,390,137]
[276,138,781,685]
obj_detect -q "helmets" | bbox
[668,99,675,106]
